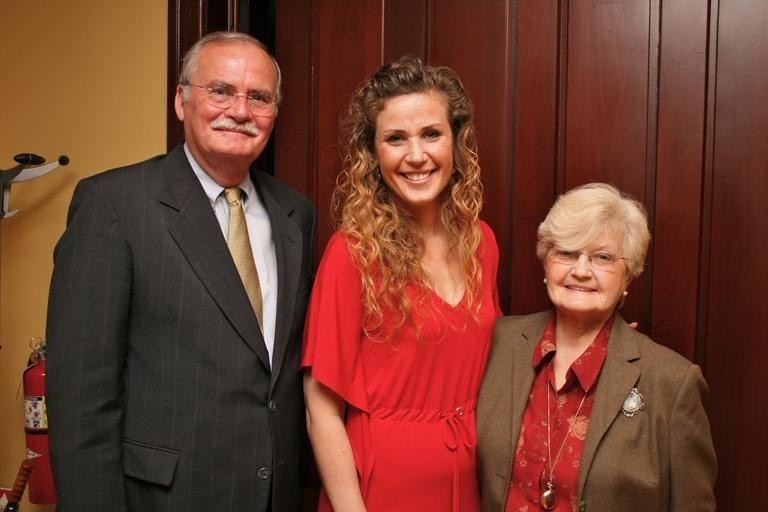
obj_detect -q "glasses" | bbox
[551,246,627,266]
[181,82,277,118]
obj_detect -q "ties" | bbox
[222,187,263,333]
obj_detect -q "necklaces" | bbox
[538,382,589,512]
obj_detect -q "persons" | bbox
[46,34,316,510]
[299,54,641,511]
[475,181,718,511]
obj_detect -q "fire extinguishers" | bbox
[23,336,56,505]
